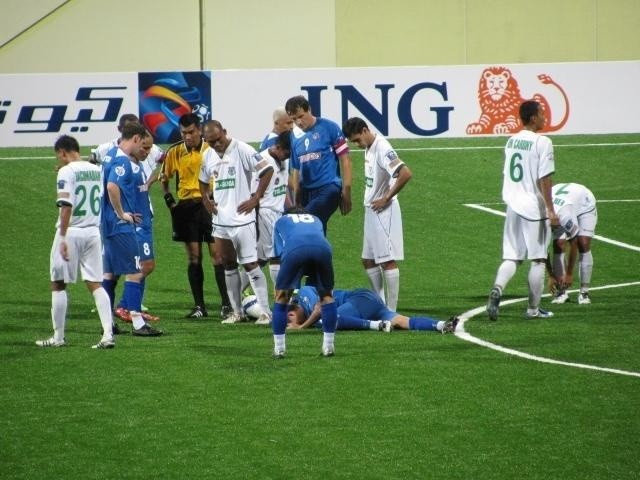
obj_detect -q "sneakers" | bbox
[220,305,231,318]
[220,313,249,324]
[551,292,569,305]
[525,307,554,319]
[185,305,208,319]
[114,307,132,322]
[131,324,164,337]
[440,316,460,335]
[112,322,130,335]
[142,312,161,322]
[254,311,273,325]
[34,337,67,348]
[378,319,392,334]
[577,292,592,305]
[486,286,502,321]
[91,337,116,348]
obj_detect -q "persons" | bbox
[35,137,115,349]
[343,118,411,312]
[547,182,598,304]
[487,101,561,322]
[287,284,458,334]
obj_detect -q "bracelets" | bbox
[60,235,65,243]
